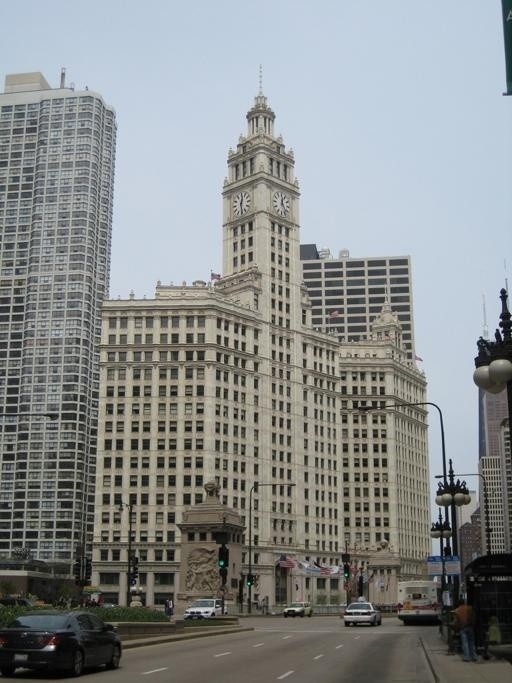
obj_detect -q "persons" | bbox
[453,598,479,662]
[57,598,100,608]
[485,617,502,662]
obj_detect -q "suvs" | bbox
[283,601,313,617]
[182,598,228,620]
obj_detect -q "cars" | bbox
[1,609,124,678]
[342,602,383,626]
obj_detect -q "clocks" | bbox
[271,190,291,217]
[232,191,252,219]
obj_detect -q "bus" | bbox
[395,580,445,626]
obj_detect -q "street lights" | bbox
[429,506,452,591]
[117,501,133,607]
[339,401,452,556]
[248,482,297,613]
[472,285,512,472]
[435,457,473,555]
[433,472,491,557]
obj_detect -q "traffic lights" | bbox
[218,547,229,567]
[134,566,139,578]
[246,573,254,585]
[344,564,349,577]
[74,558,81,580]
[85,558,93,579]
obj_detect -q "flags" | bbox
[330,310,338,319]
[211,273,220,281]
[279,555,297,568]
